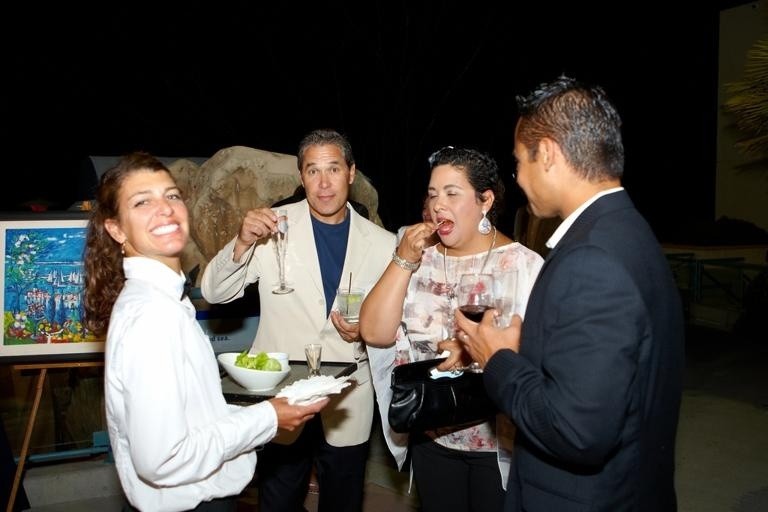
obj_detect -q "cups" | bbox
[336,289,365,323]
[492,267,519,329]
[305,344,322,373]
[457,275,492,367]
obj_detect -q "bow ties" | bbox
[180,264,199,300]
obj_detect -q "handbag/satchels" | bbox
[388,358,493,433]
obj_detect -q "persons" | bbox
[81,73,687,511]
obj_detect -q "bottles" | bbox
[265,352,289,366]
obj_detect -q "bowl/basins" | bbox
[217,351,292,390]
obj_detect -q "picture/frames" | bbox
[1,210,111,362]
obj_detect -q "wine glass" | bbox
[268,209,294,294]
